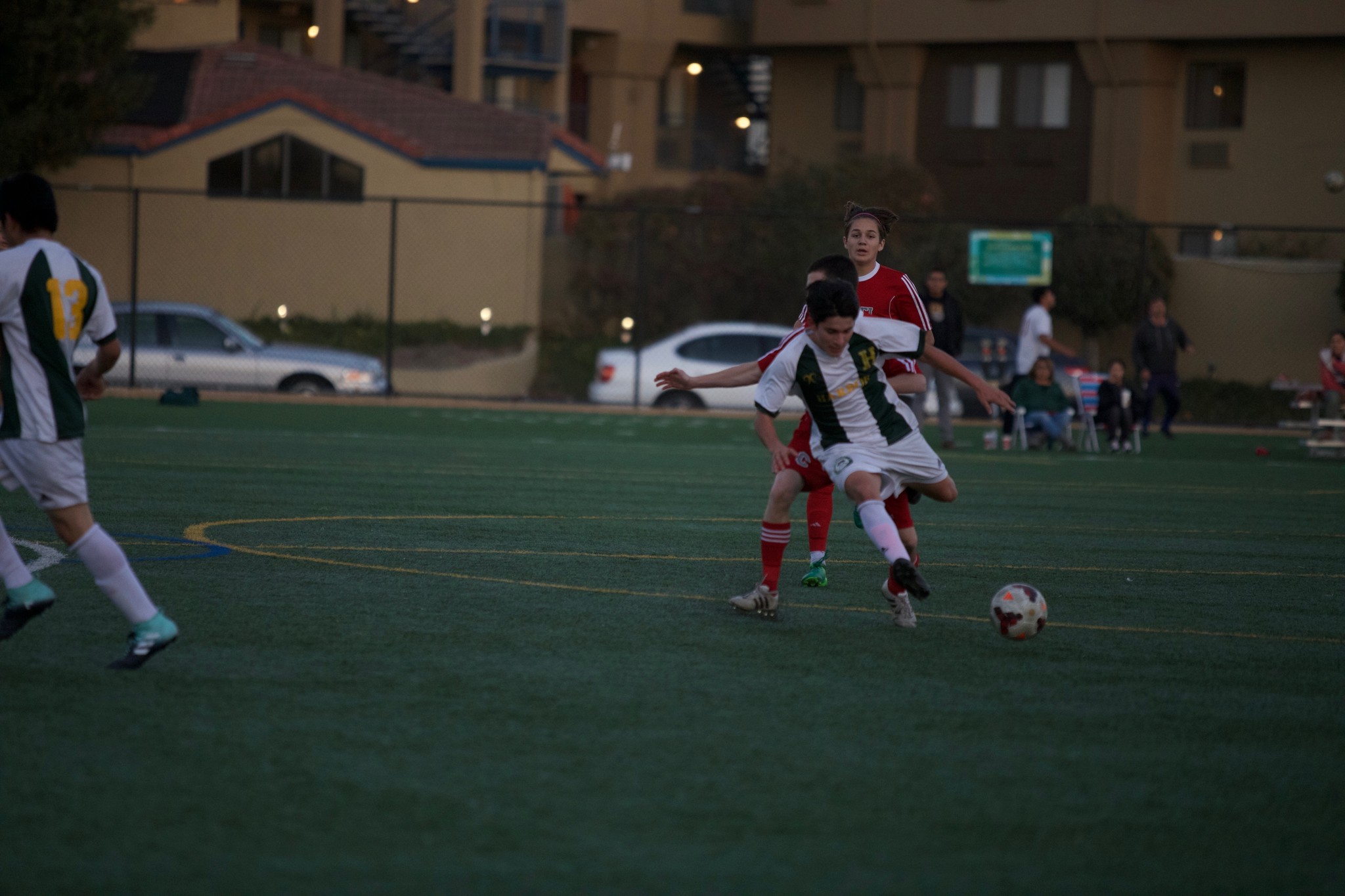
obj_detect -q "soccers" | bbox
[988,583,1048,639]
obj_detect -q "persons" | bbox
[1132,294,1197,443]
[1315,332,1344,443]
[753,277,1020,599]
[0,166,185,675]
[1093,357,1145,452]
[1002,283,1073,445]
[803,197,935,589]
[1009,353,1081,455]
[655,255,1017,619]
[909,261,968,450]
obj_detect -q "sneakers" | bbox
[108,612,179,670]
[0,577,54,640]
[800,546,830,586]
[894,558,931,600]
[725,573,778,617]
[880,580,915,627]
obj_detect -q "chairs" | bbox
[1074,372,1144,454]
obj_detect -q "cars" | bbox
[587,322,964,420]
[72,301,389,396]
